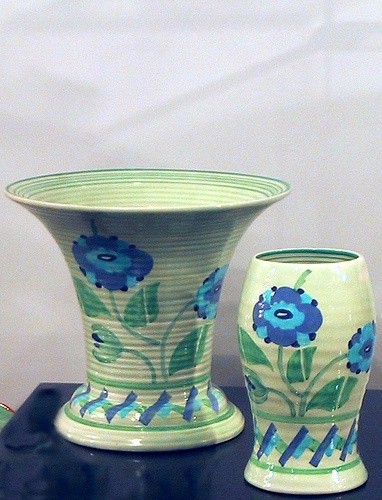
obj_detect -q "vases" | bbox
[234,246,380,497]
[0,164,291,455]
